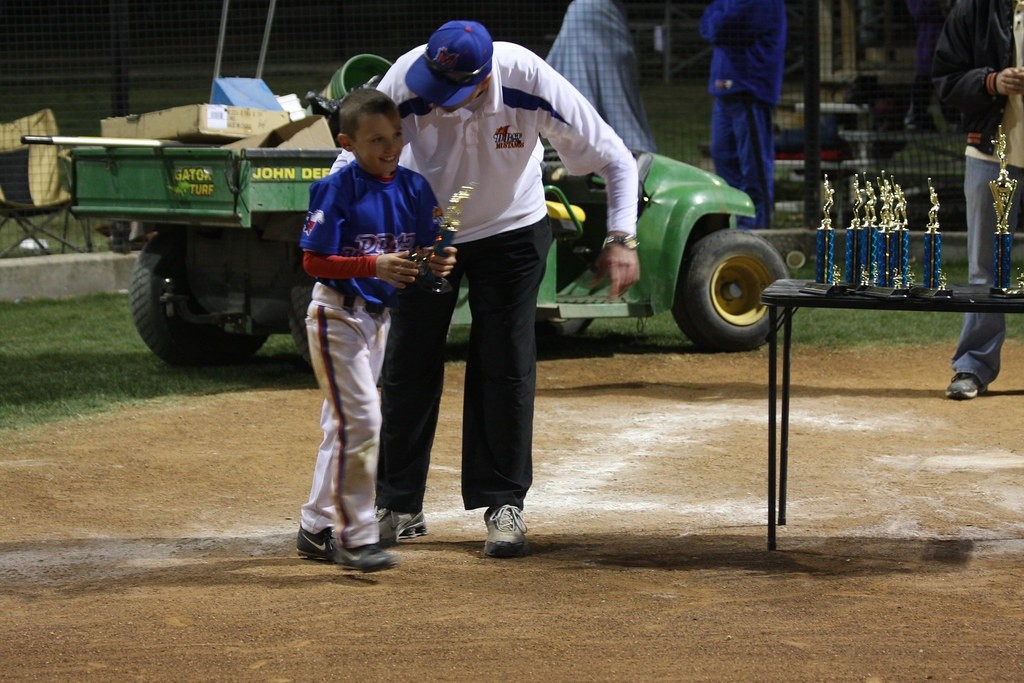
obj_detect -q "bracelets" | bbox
[601,235,640,251]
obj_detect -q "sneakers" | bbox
[484,505,527,557]
[296,522,336,560]
[374,505,427,546]
[945,373,987,398]
[335,543,399,570]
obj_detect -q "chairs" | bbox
[0,107,93,255]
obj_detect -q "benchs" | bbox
[544,183,585,235]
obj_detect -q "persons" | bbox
[839,74,909,161]
[331,20,642,557]
[930,1,1024,400]
[701,0,789,228]
[906,0,952,133]
[295,91,458,574]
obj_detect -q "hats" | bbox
[405,21,493,107]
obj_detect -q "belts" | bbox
[344,296,386,316]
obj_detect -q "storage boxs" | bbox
[101,102,289,141]
[221,114,336,150]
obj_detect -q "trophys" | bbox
[988,125,1024,298]
[910,178,953,298]
[842,168,912,298]
[801,174,847,296]
[409,182,475,295]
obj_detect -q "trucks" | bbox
[61,143,791,389]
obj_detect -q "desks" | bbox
[761,277,1024,552]
